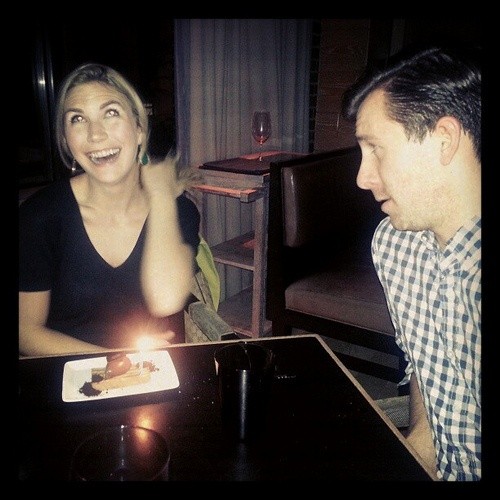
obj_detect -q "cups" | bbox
[74,427,171,500]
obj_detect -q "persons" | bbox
[337,43,480,481]
[19,63,200,358]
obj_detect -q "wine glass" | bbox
[251,112,272,162]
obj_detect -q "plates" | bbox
[61,351,180,403]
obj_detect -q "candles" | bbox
[136,334,152,371]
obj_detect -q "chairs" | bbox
[183,293,240,342]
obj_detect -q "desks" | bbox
[19,334,444,481]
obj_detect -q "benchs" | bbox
[265,147,411,397]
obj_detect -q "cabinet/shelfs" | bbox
[190,150,316,339]
